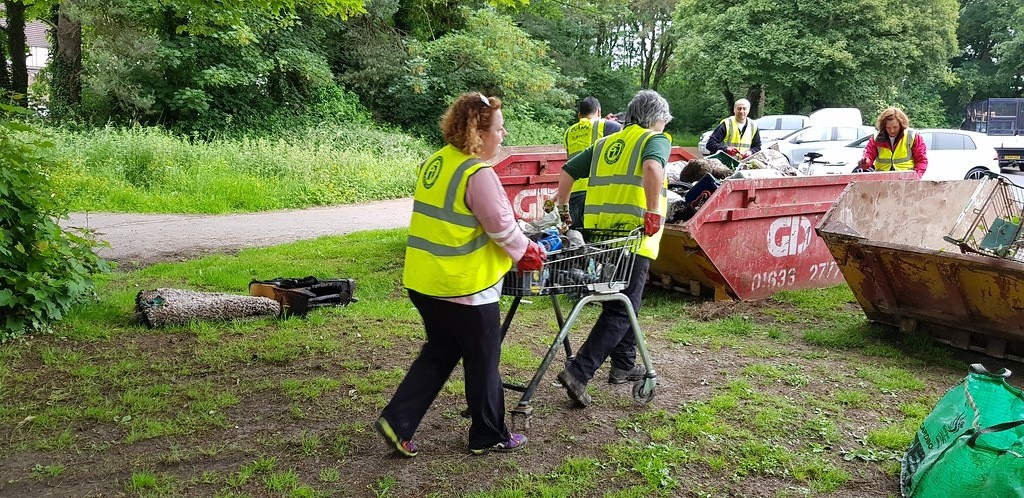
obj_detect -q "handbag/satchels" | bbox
[900,363,1024,498]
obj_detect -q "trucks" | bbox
[958,97,1024,171]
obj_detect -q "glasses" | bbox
[474,92,491,112]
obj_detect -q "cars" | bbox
[698,107,1000,182]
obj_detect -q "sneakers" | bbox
[557,365,591,406]
[608,362,647,383]
[469,433,528,454]
[375,417,419,457]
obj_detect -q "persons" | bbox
[374,92,546,458]
[858,107,928,180]
[556,88,673,409]
[706,98,762,160]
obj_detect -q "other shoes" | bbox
[580,292,602,308]
[568,293,581,303]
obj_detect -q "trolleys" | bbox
[943,170,1024,264]
[502,224,660,430]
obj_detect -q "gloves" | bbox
[517,237,547,277]
[643,211,662,237]
[727,146,739,156]
[742,150,752,159]
[556,204,572,225]
